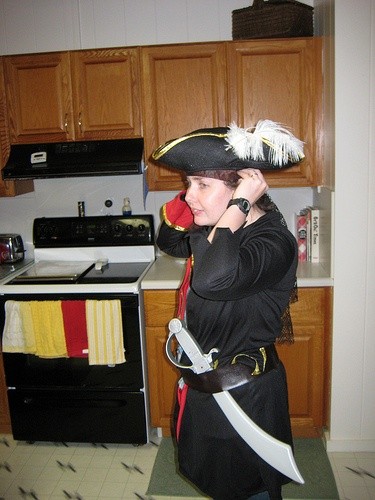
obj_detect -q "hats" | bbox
[152,118,306,175]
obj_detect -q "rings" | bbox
[251,173,258,177]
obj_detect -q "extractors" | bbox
[1,137,145,182]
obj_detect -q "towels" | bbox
[30,301,70,358]
[2,300,36,354]
[61,301,88,358]
[85,299,126,368]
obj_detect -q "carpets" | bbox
[145,437,339,500]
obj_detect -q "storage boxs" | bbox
[295,207,320,263]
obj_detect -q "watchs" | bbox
[227,198,251,217]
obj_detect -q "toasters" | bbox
[0,233,26,263]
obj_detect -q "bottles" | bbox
[122,198,131,215]
[78,201,85,217]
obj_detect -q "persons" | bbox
[148,119,305,500]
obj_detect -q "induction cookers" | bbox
[7,213,156,284]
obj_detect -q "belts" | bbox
[177,342,279,393]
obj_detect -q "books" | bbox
[295,206,319,262]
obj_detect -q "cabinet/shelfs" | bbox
[141,36,332,187]
[143,287,334,439]
[0,55,34,198]
[2,44,145,144]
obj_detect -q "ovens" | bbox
[1,286,150,448]
